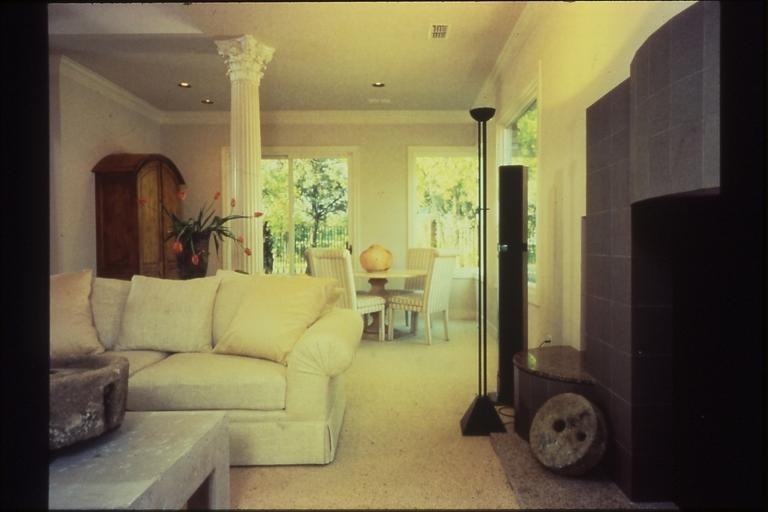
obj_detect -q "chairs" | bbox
[305,246,452,345]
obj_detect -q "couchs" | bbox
[50,302,352,467]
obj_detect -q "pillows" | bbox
[50,269,345,369]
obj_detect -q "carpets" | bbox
[489,428,644,511]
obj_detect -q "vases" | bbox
[175,249,208,279]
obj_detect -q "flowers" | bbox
[140,188,264,267]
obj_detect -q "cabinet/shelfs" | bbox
[91,151,187,280]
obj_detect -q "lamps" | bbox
[459,106,508,438]
